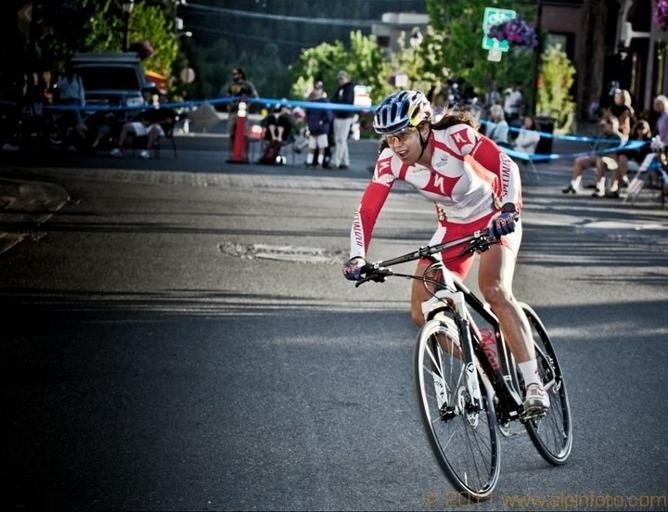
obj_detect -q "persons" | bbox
[427,76,668,200]
[259,71,357,169]
[55,60,86,126]
[107,93,175,160]
[224,66,258,145]
[340,87,553,418]
[92,95,130,151]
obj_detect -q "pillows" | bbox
[142,82,159,96]
[372,90,433,134]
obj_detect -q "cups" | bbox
[386,126,416,144]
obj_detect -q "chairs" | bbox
[110,149,120,157]
[564,179,629,197]
[304,160,348,170]
[524,383,550,408]
[140,150,149,158]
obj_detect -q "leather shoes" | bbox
[489,203,519,240]
[342,256,367,280]
